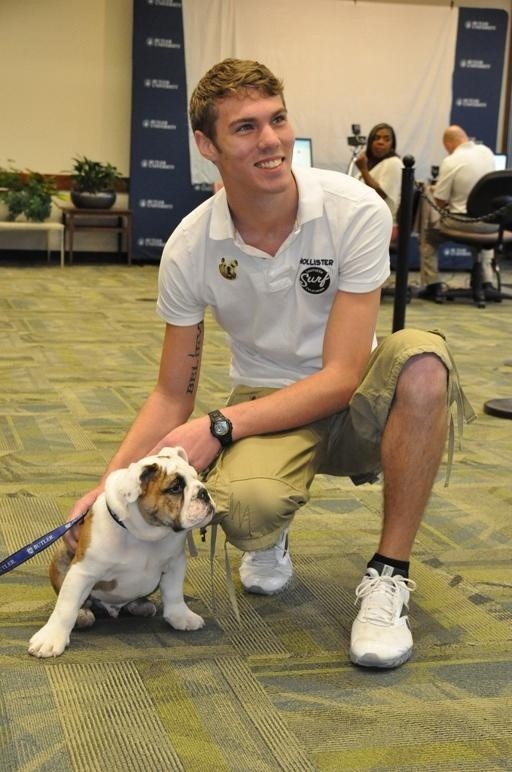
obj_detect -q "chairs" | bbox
[429,166,511,308]
[379,177,429,304]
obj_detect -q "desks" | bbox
[2,218,65,270]
[52,203,133,268]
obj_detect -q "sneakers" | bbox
[236,527,296,596]
[469,281,498,291]
[347,558,416,670]
[416,281,451,301]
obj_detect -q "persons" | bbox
[347,121,402,215]
[416,122,502,302]
[63,56,457,673]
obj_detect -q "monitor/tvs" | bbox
[292,138,313,168]
[492,153,508,170]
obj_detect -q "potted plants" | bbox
[71,155,127,206]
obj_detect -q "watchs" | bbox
[207,407,233,448]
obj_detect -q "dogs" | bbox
[26,446,217,659]
